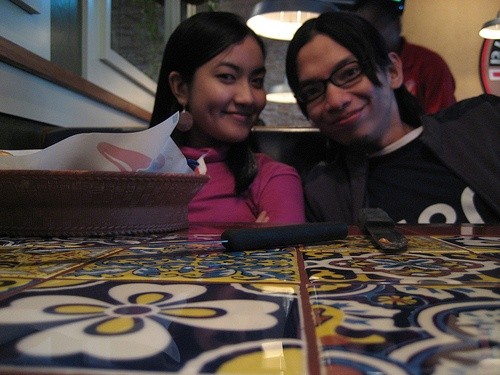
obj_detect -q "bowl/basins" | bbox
[1,170,210,233]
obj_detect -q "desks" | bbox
[0,220,500,375]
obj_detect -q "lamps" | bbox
[478,10,500,39]
[246,0,340,42]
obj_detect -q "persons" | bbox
[331,0,456,113]
[286,13,500,225]
[150,12,305,225]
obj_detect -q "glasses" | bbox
[294,60,363,103]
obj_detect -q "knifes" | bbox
[22,220,347,255]
[355,207,407,251]
